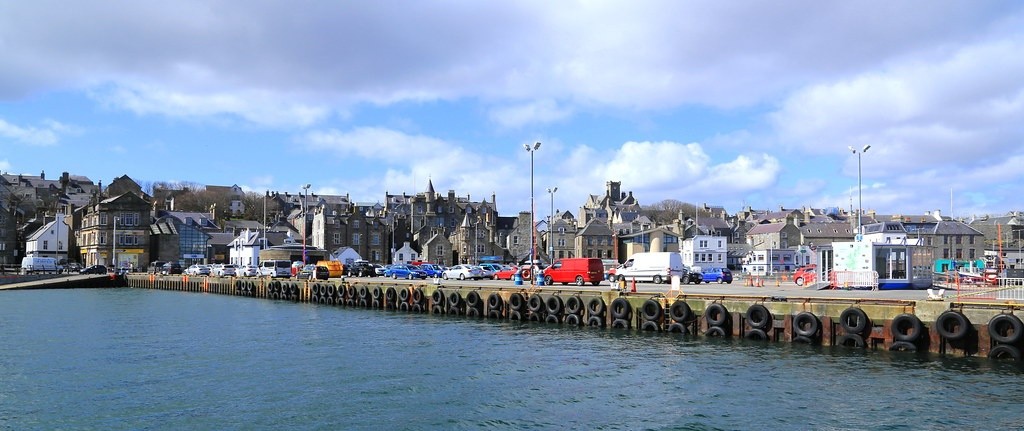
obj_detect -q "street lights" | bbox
[847,145,871,242]
[545,187,558,265]
[302,184,312,267]
[523,142,543,285]
[113,216,120,267]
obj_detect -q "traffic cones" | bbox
[749,275,755,287]
[756,275,761,287]
[630,276,637,292]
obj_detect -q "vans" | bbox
[21,256,64,274]
[544,257,605,286]
[608,251,683,284]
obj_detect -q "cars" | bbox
[701,267,733,284]
[69,262,84,272]
[79,264,107,275]
[792,264,817,286]
[146,258,518,281]
[680,268,703,284]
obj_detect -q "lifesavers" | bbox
[241,280,247,291]
[347,286,356,299]
[359,286,368,299]
[282,294,287,300]
[386,302,395,310]
[373,301,382,309]
[386,287,396,301]
[319,284,326,296]
[290,295,297,301]
[399,287,1023,362]
[273,292,279,299]
[373,286,382,300]
[282,282,289,294]
[248,291,253,297]
[327,284,335,297]
[312,283,320,295]
[242,291,247,296]
[337,285,346,298]
[235,290,241,296]
[290,282,298,294]
[274,280,281,292]
[235,280,241,290]
[311,295,369,308]
[247,281,254,291]
[267,282,274,293]
